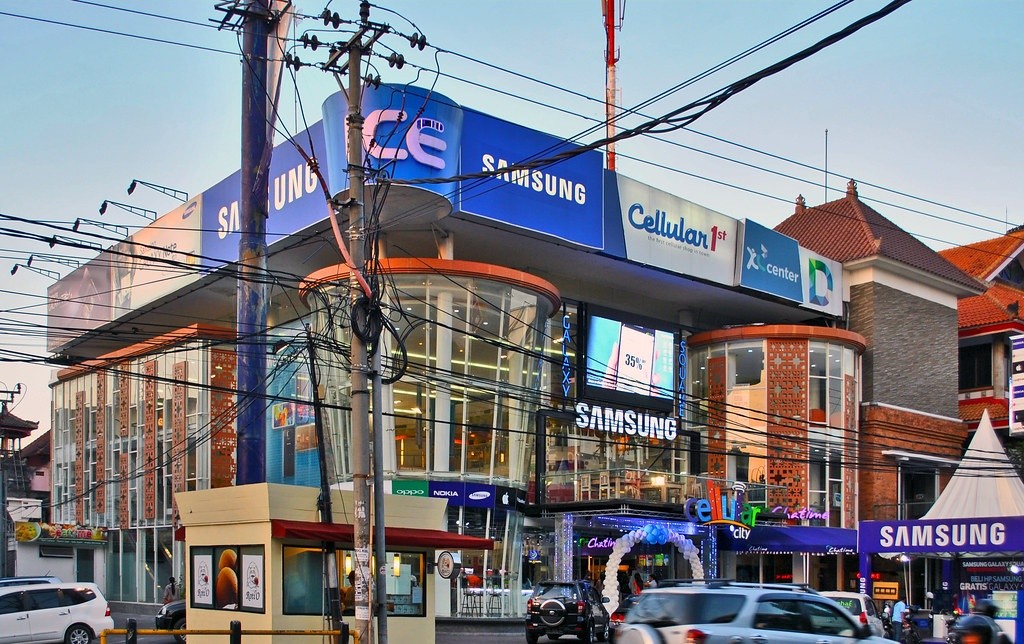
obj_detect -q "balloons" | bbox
[601,524,705,617]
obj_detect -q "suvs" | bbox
[617,579,901,644]
[525,579,610,644]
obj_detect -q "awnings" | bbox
[271,519,494,551]
[175,526,185,541]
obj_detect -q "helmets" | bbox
[952,615,991,633]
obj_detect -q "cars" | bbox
[816,591,889,637]
[0,575,61,587]
[609,594,639,644]
[155,599,186,644]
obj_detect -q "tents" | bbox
[879,409,1024,610]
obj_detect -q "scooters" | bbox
[944,613,955,644]
[902,609,922,644]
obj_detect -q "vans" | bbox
[0,582,114,644]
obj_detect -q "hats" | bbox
[346,570,355,579]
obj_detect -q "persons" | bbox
[340,571,355,616]
[461,568,469,588]
[958,599,1013,644]
[643,574,658,587]
[585,570,632,600]
[633,573,643,595]
[891,596,907,641]
[164,577,180,605]
[493,567,506,588]
[952,592,975,612]
